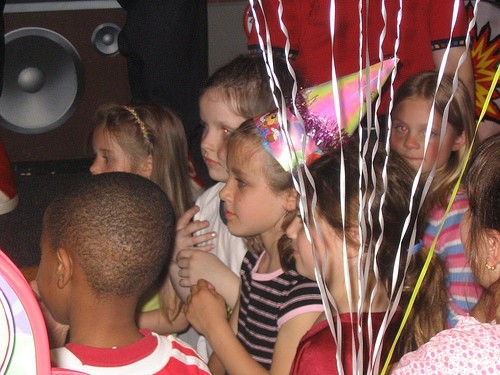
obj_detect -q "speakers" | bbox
[0,0,134,165]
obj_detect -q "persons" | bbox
[392,133,500,375]
[244,0,475,115]
[30,52,479,375]
[37,172,213,375]
[464,0,500,144]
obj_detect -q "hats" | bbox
[254,57,400,175]
